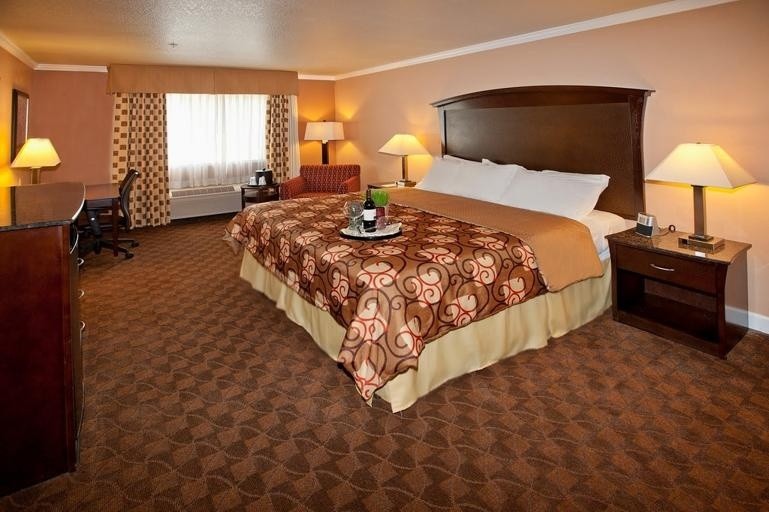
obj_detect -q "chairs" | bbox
[79,168,139,259]
[280,164,360,200]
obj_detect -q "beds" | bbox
[222,85,655,414]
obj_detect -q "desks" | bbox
[82,184,120,257]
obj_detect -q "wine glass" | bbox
[341,201,363,229]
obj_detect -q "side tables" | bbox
[241,183,280,209]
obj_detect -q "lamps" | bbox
[304,120,344,164]
[645,142,757,250]
[378,134,430,186]
[8,138,61,183]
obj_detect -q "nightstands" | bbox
[603,225,753,359]
[368,182,416,190]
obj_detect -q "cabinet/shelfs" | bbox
[0,182,85,496]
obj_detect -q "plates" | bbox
[341,226,402,239]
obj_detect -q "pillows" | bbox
[416,154,610,220]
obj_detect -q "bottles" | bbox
[363,189,377,232]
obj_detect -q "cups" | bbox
[250,176,267,186]
[375,207,385,220]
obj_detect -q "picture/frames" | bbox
[11,89,29,163]
